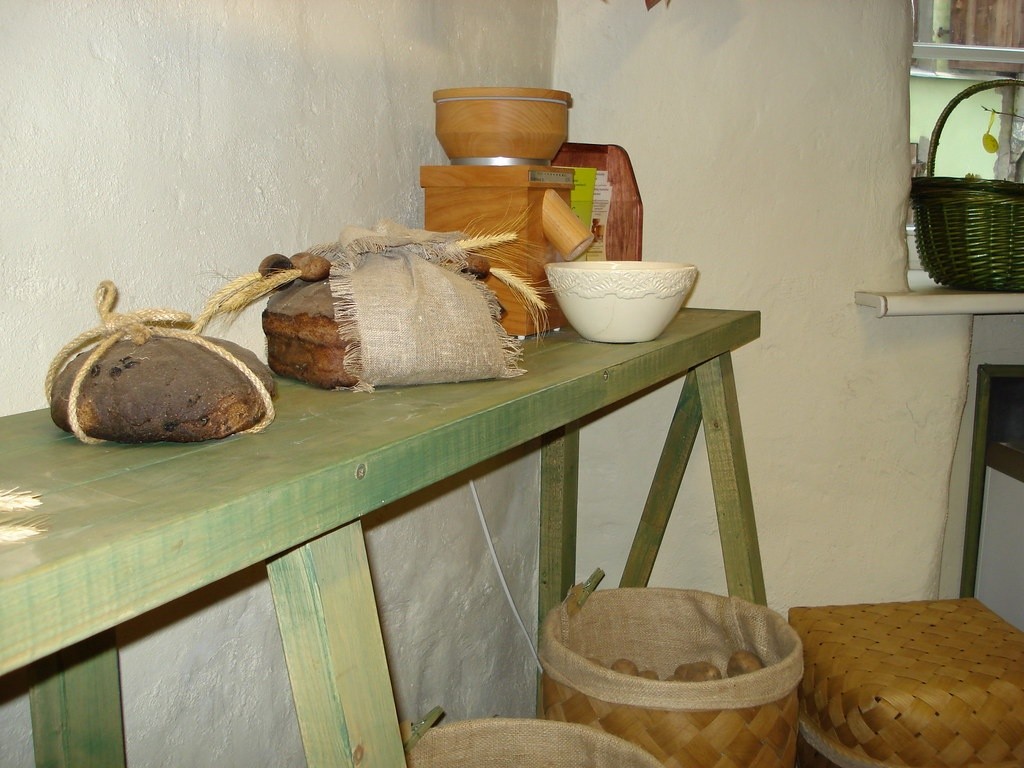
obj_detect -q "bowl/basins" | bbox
[543,260,698,343]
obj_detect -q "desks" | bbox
[1,308,766,768]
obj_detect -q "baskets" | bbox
[911,79,1024,294]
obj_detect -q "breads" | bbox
[50,333,277,444]
[260,256,504,390]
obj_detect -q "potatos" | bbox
[586,651,762,683]
